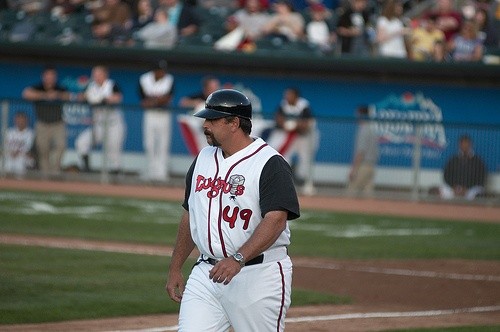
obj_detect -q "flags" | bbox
[177,114,297,170]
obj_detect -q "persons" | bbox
[0,0,199,47]
[437,135,485,201]
[0,67,66,174]
[224,0,500,64]
[348,106,377,199]
[84,66,125,185]
[138,60,176,183]
[166,77,319,332]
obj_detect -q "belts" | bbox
[207,255,265,267]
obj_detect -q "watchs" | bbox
[231,253,245,268]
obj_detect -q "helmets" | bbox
[194,88,254,121]
